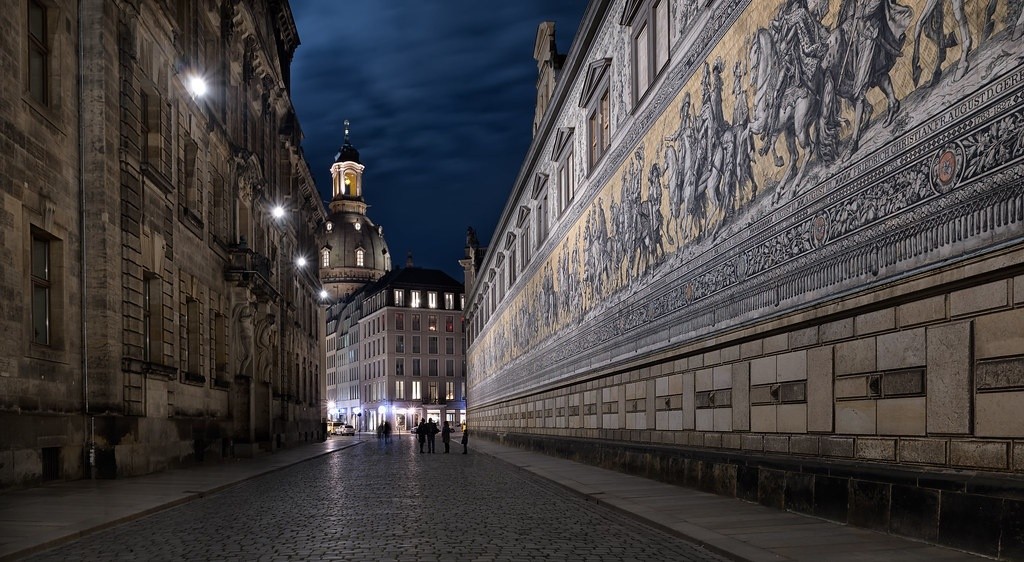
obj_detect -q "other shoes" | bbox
[462,452,467,454]
[432,452,435,453]
[420,450,425,453]
[443,451,449,453]
[428,452,431,453]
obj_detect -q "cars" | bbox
[411,424,419,433]
[335,424,355,436]
[449,424,463,433]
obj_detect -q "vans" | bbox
[326,421,344,436]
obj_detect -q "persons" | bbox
[322,418,327,442]
[231,302,254,376]
[442,421,450,454]
[418,418,436,453]
[256,313,275,382]
[384,422,391,443]
[461,430,468,454]
